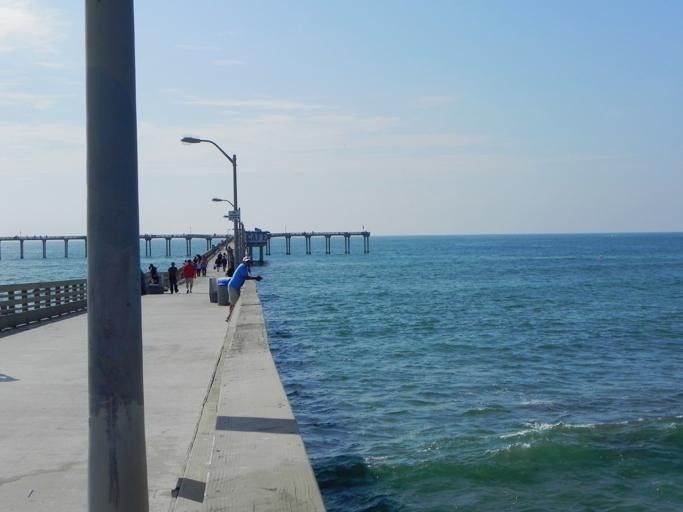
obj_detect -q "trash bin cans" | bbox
[218,278,231,305]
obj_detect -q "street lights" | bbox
[179,135,239,273]
[210,198,248,265]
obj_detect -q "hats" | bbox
[243,256,251,262]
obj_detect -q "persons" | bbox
[141,271,146,295]
[183,259,195,293]
[150,264,159,284]
[183,254,208,277]
[168,262,179,294]
[213,243,232,272]
[225,256,263,322]
